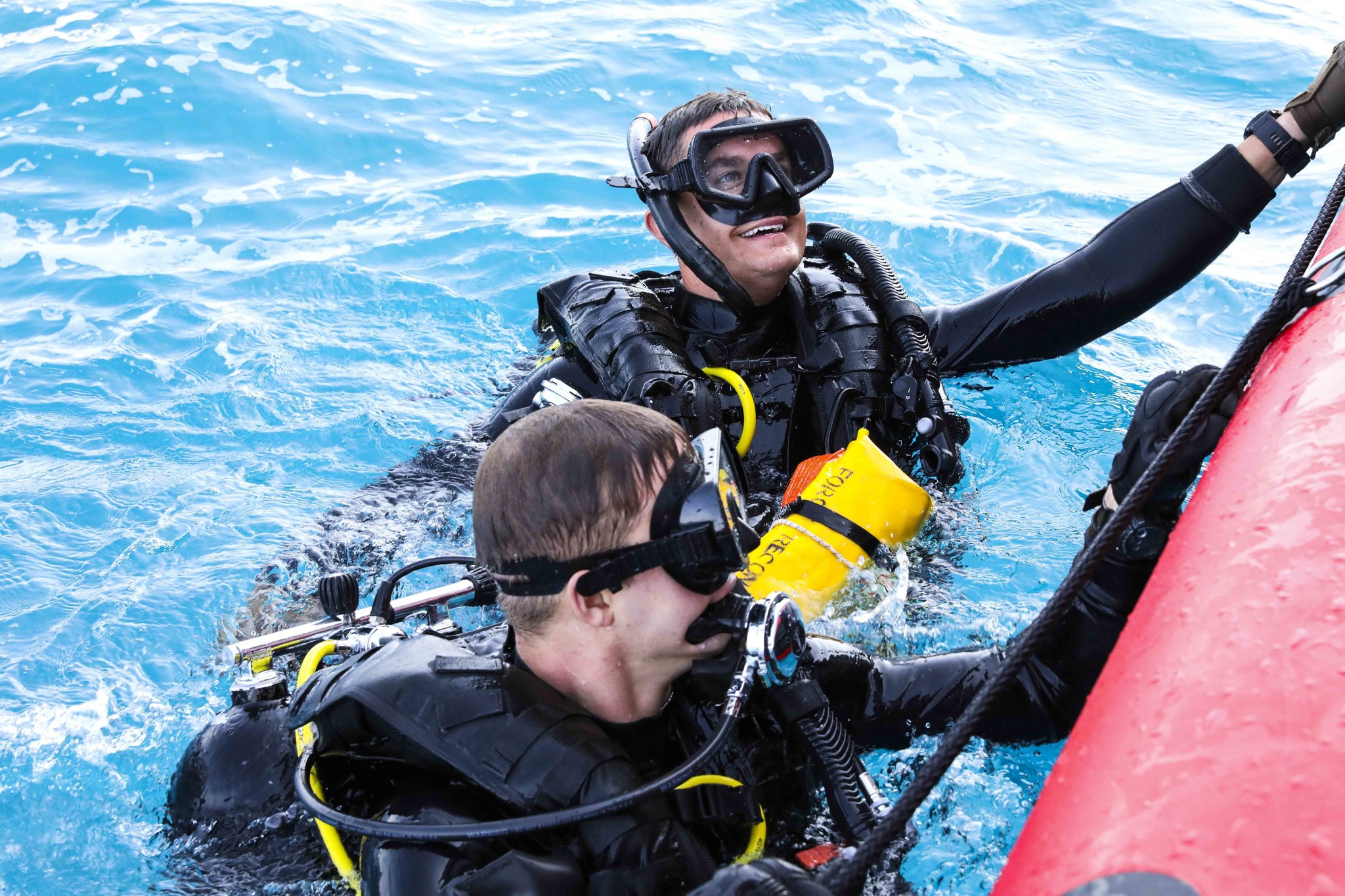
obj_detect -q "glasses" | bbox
[496,427,761,597]
[643,114,835,229]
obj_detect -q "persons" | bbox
[351,365,1222,896]
[479,36,1345,507]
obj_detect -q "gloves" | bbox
[685,856,836,896]
[1109,361,1241,513]
[1287,37,1345,150]
